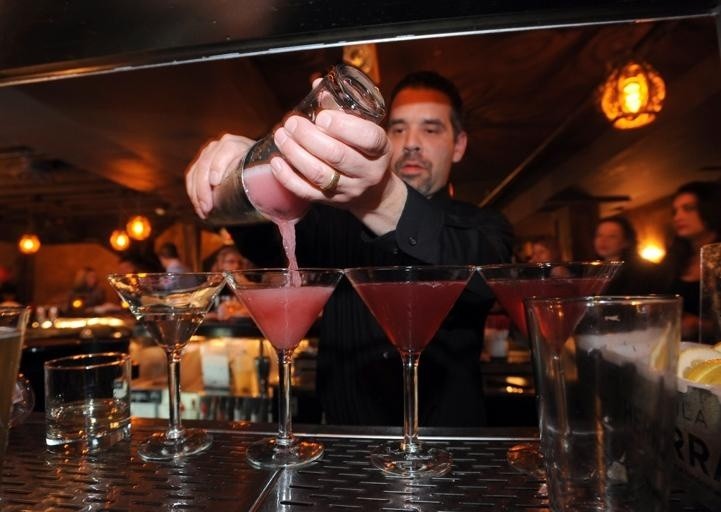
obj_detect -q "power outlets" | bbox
[18,231,41,254]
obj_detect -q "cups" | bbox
[245,59,388,223]
[43,350,131,459]
[523,292,684,507]
[206,138,309,227]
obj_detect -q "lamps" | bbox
[110,229,132,252]
[126,213,153,240]
[599,50,666,131]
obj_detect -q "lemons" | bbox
[677,341,721,390]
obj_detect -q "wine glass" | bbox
[343,264,481,481]
[105,270,232,461]
[475,258,626,482]
[221,266,345,470]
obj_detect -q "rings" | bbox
[321,171,340,194]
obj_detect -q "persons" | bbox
[0,269,20,307]
[186,68,518,427]
[70,240,259,318]
[517,179,721,348]
